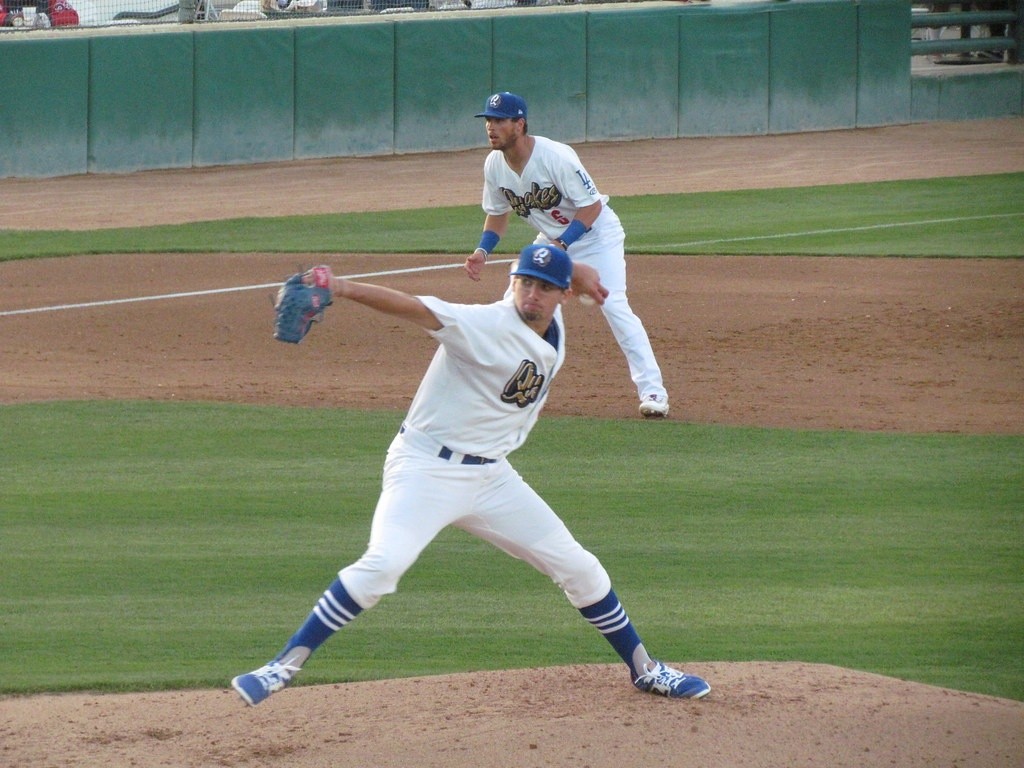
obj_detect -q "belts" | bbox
[398,427,497,465]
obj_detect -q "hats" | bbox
[509,244,573,288]
[474,91,529,118]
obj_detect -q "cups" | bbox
[23,7,36,27]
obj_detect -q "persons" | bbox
[925,0,1009,64]
[230,243,709,708]
[463,92,669,418]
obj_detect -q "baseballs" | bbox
[579,291,603,308]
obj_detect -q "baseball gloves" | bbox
[268,263,337,345]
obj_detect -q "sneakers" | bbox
[640,394,669,416]
[632,661,711,700]
[231,660,302,707]
